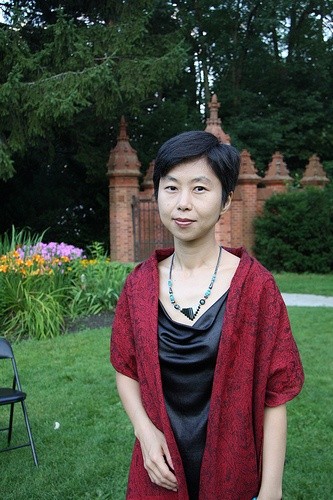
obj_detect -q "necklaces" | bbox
[168,244,221,320]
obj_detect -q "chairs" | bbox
[0,338,38,467]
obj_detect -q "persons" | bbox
[110,131,305,500]
